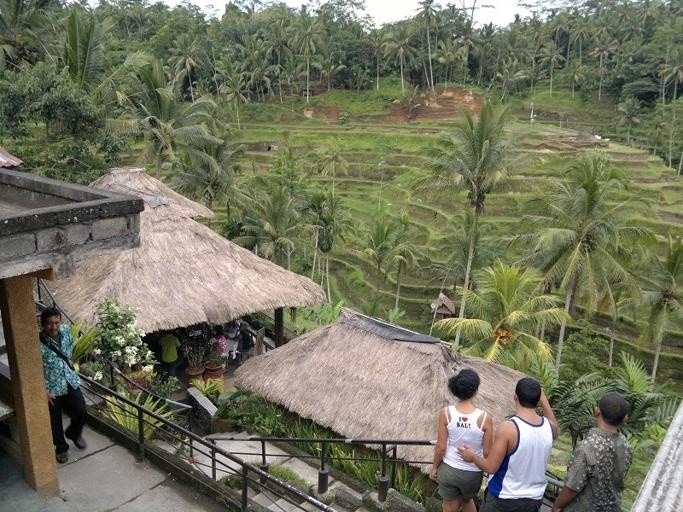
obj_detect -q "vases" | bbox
[185,367,224,395]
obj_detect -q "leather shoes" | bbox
[64,426,88,450]
[55,443,69,463]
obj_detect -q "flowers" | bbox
[181,338,226,369]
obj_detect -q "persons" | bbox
[39,307,88,464]
[158,319,254,382]
[429,370,633,512]
